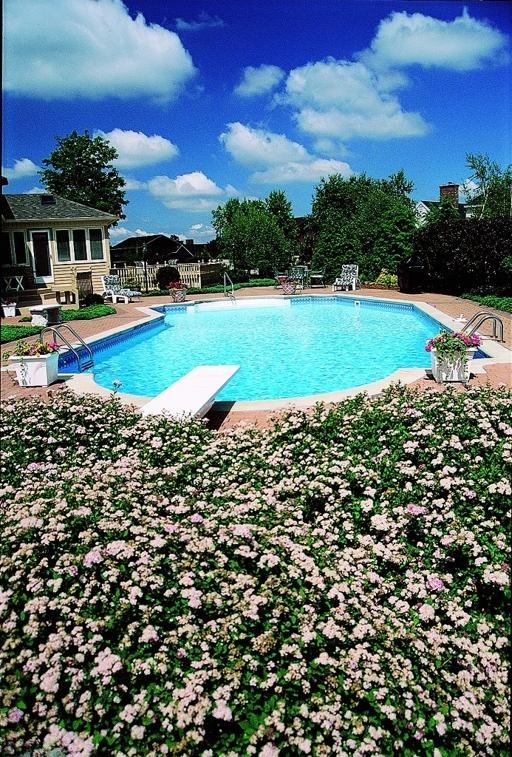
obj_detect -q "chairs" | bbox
[272,266,326,288]
[332,265,361,291]
[101,275,142,304]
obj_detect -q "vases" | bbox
[2,302,16,318]
[427,340,478,383]
[169,288,187,302]
[282,285,296,295]
[9,351,58,387]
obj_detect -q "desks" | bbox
[5,276,25,291]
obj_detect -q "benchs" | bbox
[29,304,63,327]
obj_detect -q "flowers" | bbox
[3,338,60,357]
[2,297,17,305]
[166,281,187,289]
[426,330,480,372]
